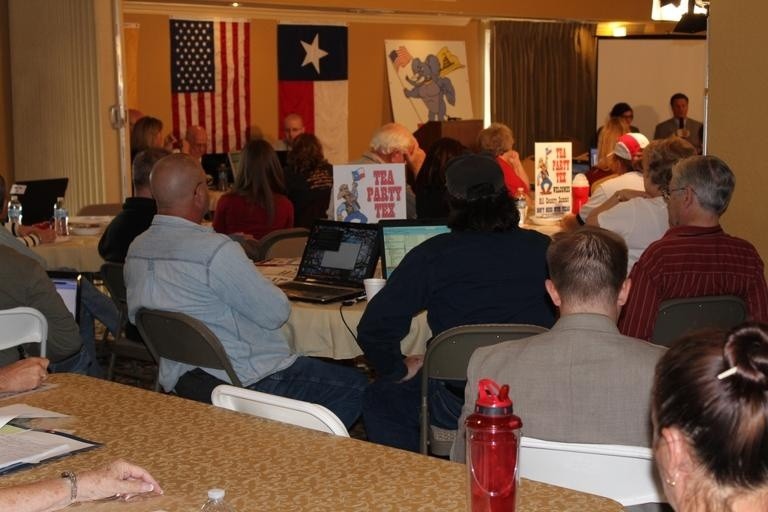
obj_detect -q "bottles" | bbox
[462,378,523,511]
[514,187,527,229]
[8,196,22,226]
[54,197,69,236]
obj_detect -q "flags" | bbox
[169,18,251,155]
[389,45,413,72]
[276,23,350,163]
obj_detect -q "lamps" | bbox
[651,0,708,31]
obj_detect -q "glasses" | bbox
[662,187,698,199]
[622,115,632,118]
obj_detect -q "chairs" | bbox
[259,227,310,263]
[208,385,347,437]
[99,262,165,392]
[130,307,242,400]
[421,321,551,457]
[517,434,667,510]
[644,295,748,346]
[1,306,49,373]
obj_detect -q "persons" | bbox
[212,138,295,240]
[653,93,703,150]
[584,136,698,279]
[181,125,216,186]
[564,131,650,229]
[0,242,96,376]
[97,146,173,343]
[585,117,632,184]
[130,115,173,161]
[281,112,306,168]
[325,123,426,220]
[448,224,674,512]
[0,221,123,338]
[0,457,165,512]
[123,152,370,431]
[648,321,768,512]
[0,356,51,393]
[597,102,640,138]
[617,154,767,343]
[356,154,560,453]
[477,122,531,200]
[0,174,57,273]
[282,131,333,228]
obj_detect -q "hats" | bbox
[607,133,650,160]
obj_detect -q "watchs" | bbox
[60,470,78,501]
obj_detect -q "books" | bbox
[332,163,408,224]
[534,141,573,218]
[1,402,104,474]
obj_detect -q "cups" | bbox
[364,279,385,301]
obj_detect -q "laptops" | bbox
[275,218,381,304]
[227,150,242,182]
[377,218,452,281]
[6,177,69,227]
[588,147,598,169]
[274,149,289,168]
[200,152,233,185]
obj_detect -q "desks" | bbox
[250,255,435,365]
[0,371,629,512]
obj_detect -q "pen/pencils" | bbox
[16,345,32,358]
